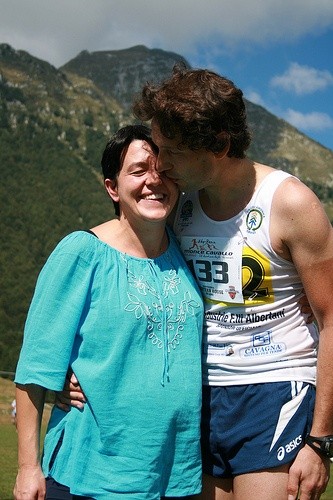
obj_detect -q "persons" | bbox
[44,66,333,500]
[13,123,213,500]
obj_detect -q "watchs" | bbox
[305,432,333,457]
